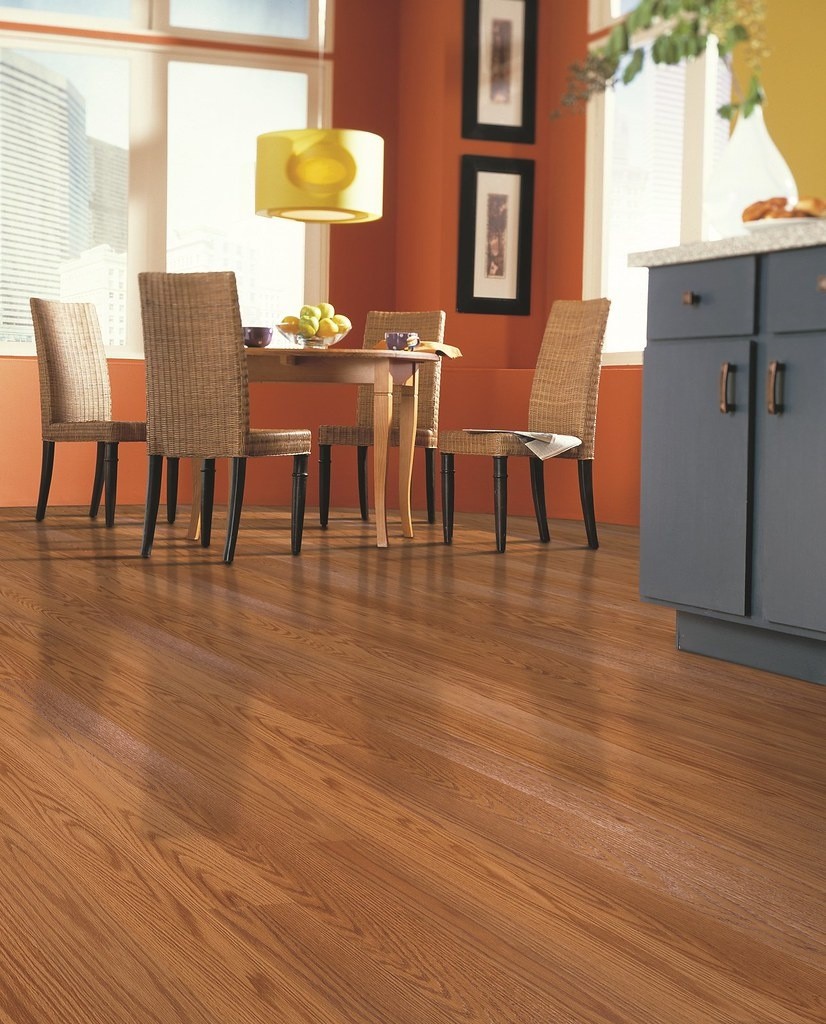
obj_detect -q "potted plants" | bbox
[545,0,826,210]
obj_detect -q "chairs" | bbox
[29,296,180,528]
[440,298,610,552]
[139,272,314,564]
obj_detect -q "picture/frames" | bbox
[462,0,538,144]
[456,153,535,317]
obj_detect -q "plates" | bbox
[741,217,820,232]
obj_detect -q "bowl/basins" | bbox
[275,324,352,350]
[384,332,410,350]
[242,327,273,347]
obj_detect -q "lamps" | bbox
[256,127,385,224]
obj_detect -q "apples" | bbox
[282,302,351,337]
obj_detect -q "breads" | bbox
[742,197,826,222]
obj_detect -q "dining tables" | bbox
[187,344,438,548]
[318,311,446,530]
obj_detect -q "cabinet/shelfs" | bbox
[641,241,826,644]
